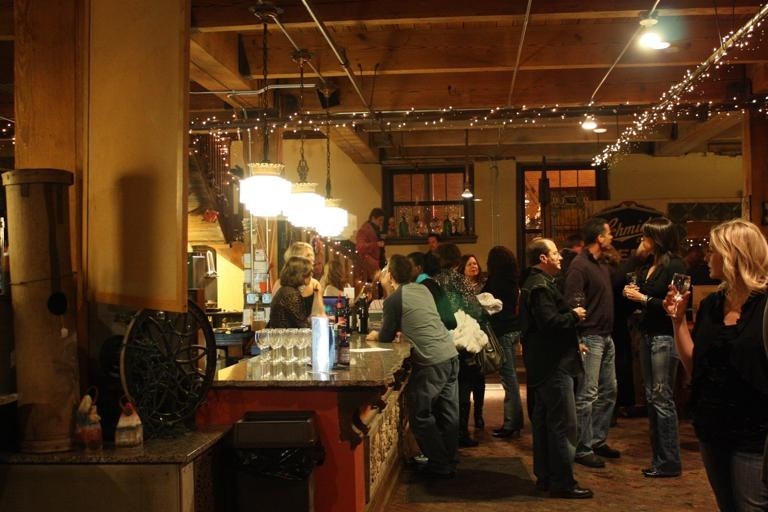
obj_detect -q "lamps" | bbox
[239,0,294,217]
[283,48,325,228]
[638,11,671,50]
[461,129,473,198]
[592,125,607,134]
[581,106,598,130]
[316,83,349,237]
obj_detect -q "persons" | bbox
[265,257,316,328]
[482,247,524,438]
[662,219,767,512]
[422,234,442,276]
[319,260,356,297]
[598,245,638,419]
[518,237,593,500]
[562,218,621,469]
[559,230,584,272]
[364,254,460,478]
[271,242,326,324]
[455,255,487,447]
[623,216,688,478]
[435,244,487,330]
[407,252,457,331]
[356,207,385,299]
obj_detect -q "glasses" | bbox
[550,251,560,259]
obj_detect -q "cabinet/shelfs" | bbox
[1,423,237,510]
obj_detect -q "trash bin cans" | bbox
[234,410,324,512]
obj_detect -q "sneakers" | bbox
[593,444,621,459]
[575,453,605,467]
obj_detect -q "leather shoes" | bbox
[409,469,434,482]
[641,469,655,474]
[425,476,470,490]
[535,483,551,491]
[643,467,676,478]
[559,486,593,499]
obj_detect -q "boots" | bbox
[458,402,479,447]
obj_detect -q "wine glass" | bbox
[629,273,637,287]
[574,292,586,320]
[578,350,588,360]
[256,328,312,367]
[381,234,387,241]
[666,273,691,318]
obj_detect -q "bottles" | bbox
[355,299,369,335]
[336,295,350,332]
[389,217,397,242]
[409,215,426,239]
[444,215,452,237]
[242,284,252,326]
[337,330,351,365]
[399,216,408,240]
[430,215,443,236]
[261,278,272,321]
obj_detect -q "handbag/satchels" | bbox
[465,321,508,376]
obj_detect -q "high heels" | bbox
[492,426,503,433]
[492,429,520,439]
[474,414,485,428]
